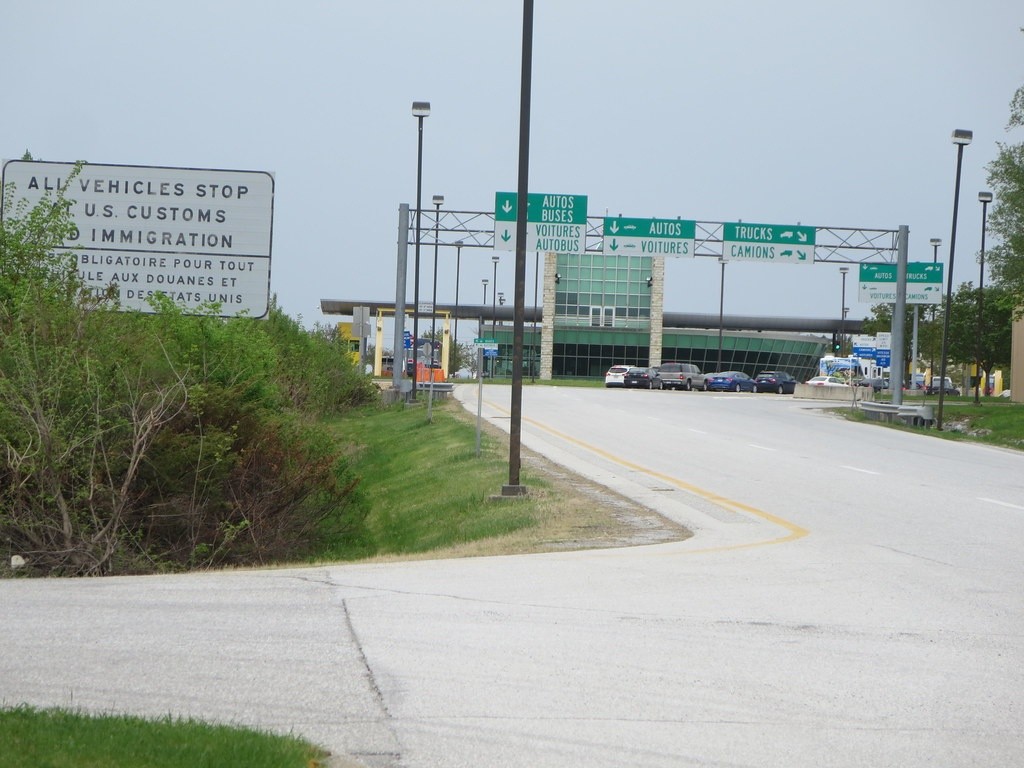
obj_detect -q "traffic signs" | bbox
[494,191,586,256]
[858,262,942,304]
[604,219,695,258]
[723,223,816,266]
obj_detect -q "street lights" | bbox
[937,128,972,430]
[428,196,506,378]
[839,267,850,337]
[716,257,731,370]
[974,191,993,408]
[929,238,943,392]
[410,101,430,403]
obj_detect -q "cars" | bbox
[710,370,758,393]
[624,367,663,390]
[805,372,960,398]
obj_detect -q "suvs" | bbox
[754,370,800,394]
[605,364,633,388]
[659,363,709,393]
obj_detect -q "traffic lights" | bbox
[834,339,841,355]
